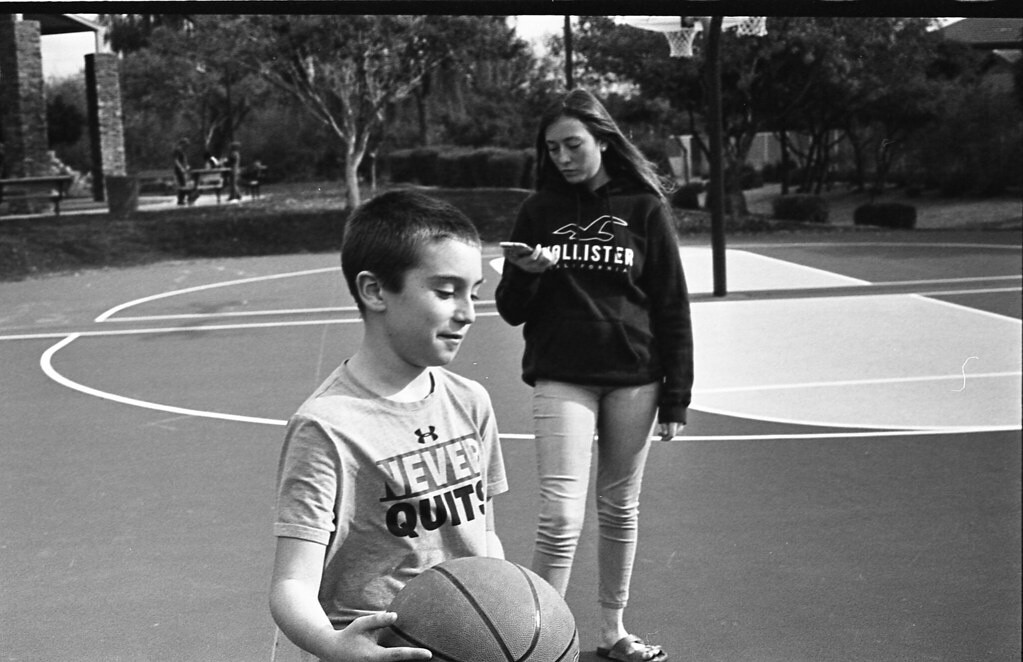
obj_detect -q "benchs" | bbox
[179,168,231,208]
[1,175,76,219]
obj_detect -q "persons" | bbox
[269,188,511,662]
[171,138,196,206]
[495,87,696,662]
[226,140,245,202]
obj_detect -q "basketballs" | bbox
[376,554,582,662]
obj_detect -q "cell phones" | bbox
[499,240,535,257]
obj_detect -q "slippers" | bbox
[596,634,667,662]
